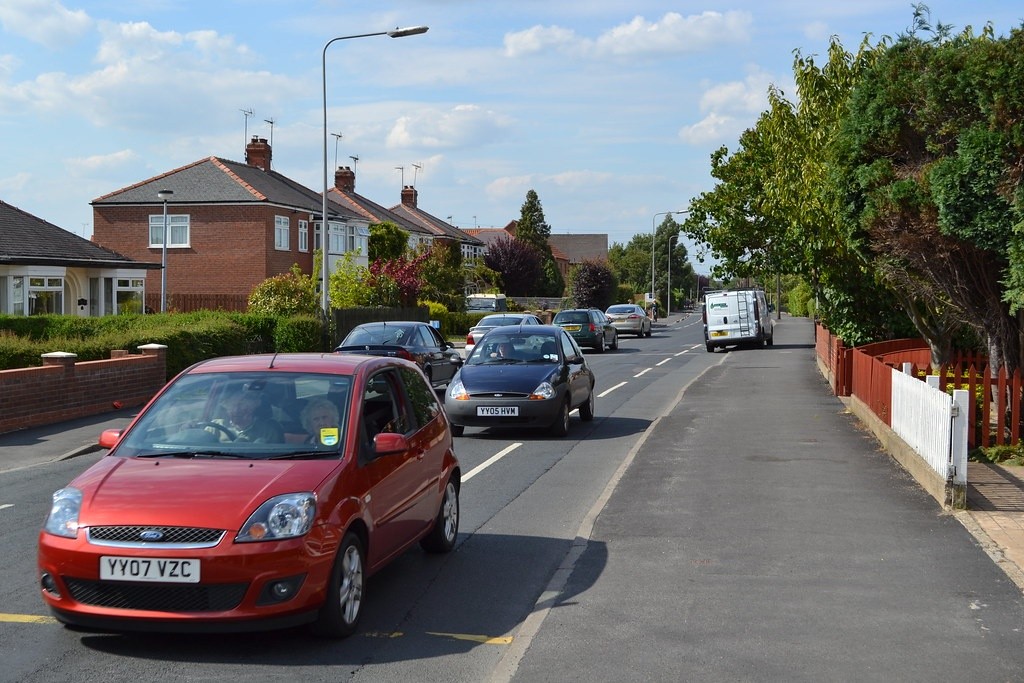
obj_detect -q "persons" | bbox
[180,388,281,444]
[653,299,658,322]
[301,398,341,448]
[489,342,514,357]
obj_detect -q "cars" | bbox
[444,323,596,440]
[334,321,463,390]
[35,352,464,638]
[464,313,545,358]
[604,304,652,338]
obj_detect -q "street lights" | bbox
[651,210,691,301]
[320,23,430,311]
[157,187,174,314]
[667,233,693,315]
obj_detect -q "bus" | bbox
[465,293,506,314]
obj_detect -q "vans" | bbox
[702,287,774,353]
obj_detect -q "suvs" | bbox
[551,307,618,352]
